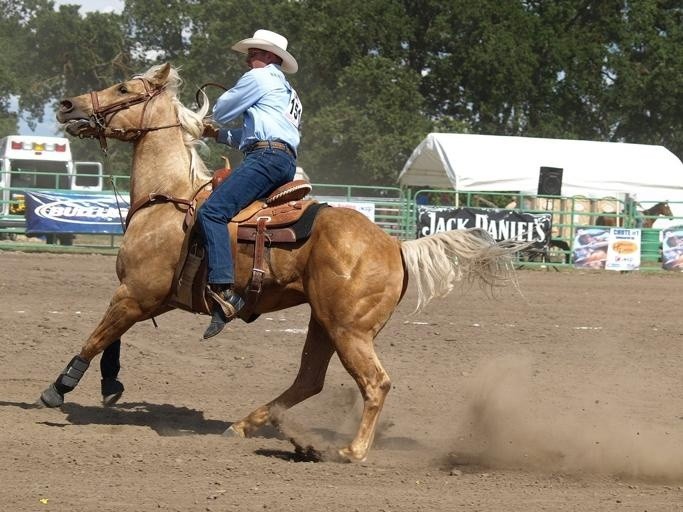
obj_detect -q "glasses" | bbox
[248,50,263,58]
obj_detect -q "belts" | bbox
[249,141,295,154]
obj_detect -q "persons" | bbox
[418,186,430,205]
[663,235,683,271]
[572,234,607,270]
[197,27,304,338]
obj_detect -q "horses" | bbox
[32,61,534,467]
[594,199,673,228]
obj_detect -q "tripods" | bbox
[518,199,560,273]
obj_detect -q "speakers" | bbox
[538,167,563,199]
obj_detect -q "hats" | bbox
[232,29,299,74]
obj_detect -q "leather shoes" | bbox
[204,290,246,339]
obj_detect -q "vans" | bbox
[0,135,102,246]
